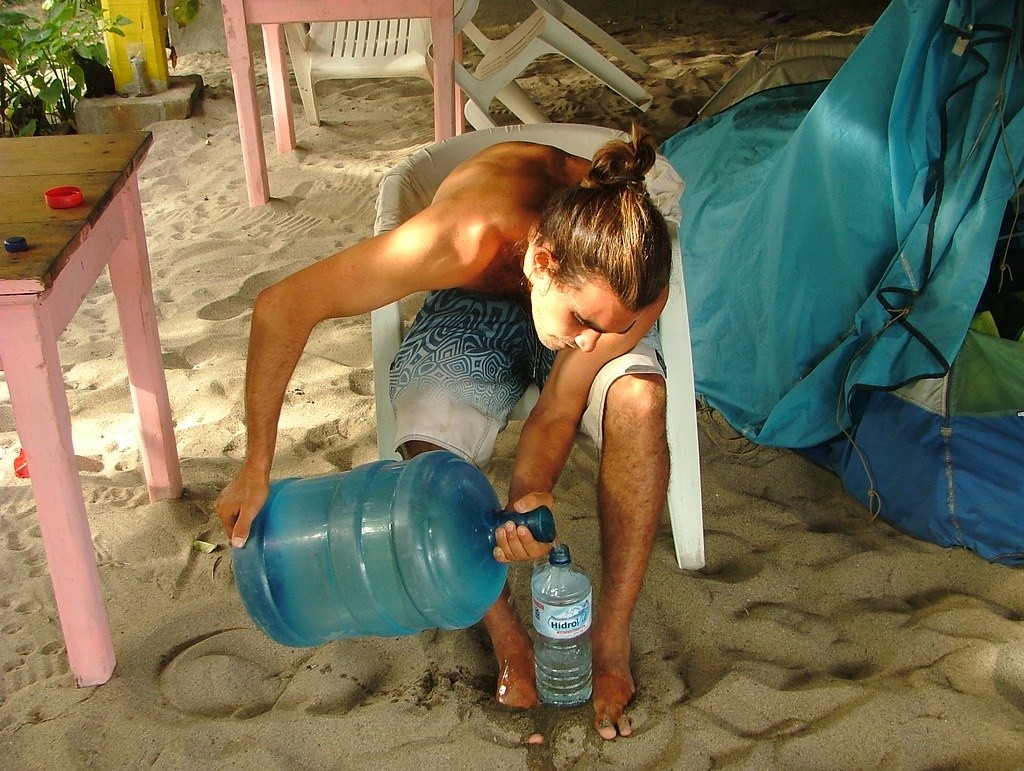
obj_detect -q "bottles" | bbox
[229,450,555,647]
[531,544,593,706]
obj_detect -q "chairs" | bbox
[283,0,653,130]
[371,123,705,569]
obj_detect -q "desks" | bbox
[221,0,464,205]
[0,131,182,687]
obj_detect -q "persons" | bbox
[217,122,671,743]
